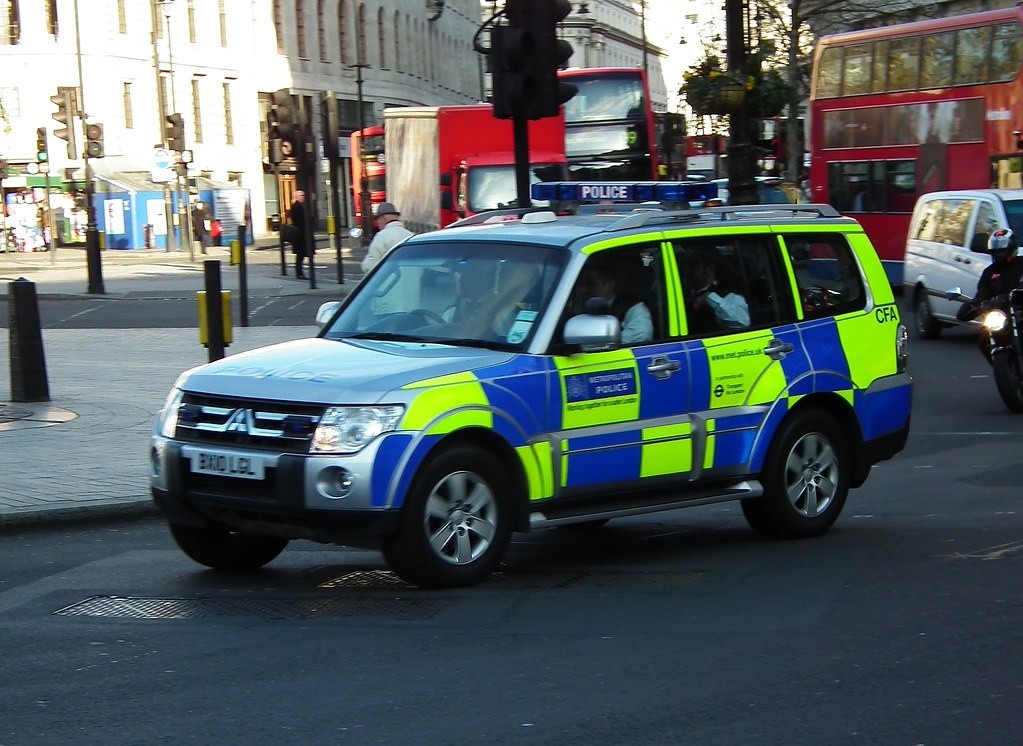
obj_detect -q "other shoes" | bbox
[297,274,304,278]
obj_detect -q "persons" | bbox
[290,191,315,279]
[362,203,425,320]
[957,228,1023,363]
[443,249,749,351]
[192,202,209,254]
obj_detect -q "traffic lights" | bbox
[270,87,295,136]
[36,128,49,174]
[50,90,77,161]
[164,113,183,152]
[86,123,105,159]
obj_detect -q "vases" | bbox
[686,86,745,113]
[745,89,787,116]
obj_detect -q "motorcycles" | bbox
[946,286,1023,413]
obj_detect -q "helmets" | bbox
[988,228,1018,263]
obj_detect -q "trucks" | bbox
[384,104,569,235]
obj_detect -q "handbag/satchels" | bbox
[283,223,303,244]
[218,226,224,232]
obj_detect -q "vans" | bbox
[902,189,1023,341]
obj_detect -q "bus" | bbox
[558,67,657,182]
[653,111,687,180]
[351,126,385,233]
[809,6,1023,287]
[686,134,729,181]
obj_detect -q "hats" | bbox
[373,202,400,220]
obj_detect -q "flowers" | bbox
[678,55,787,111]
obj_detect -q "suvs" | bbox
[146,176,915,594]
